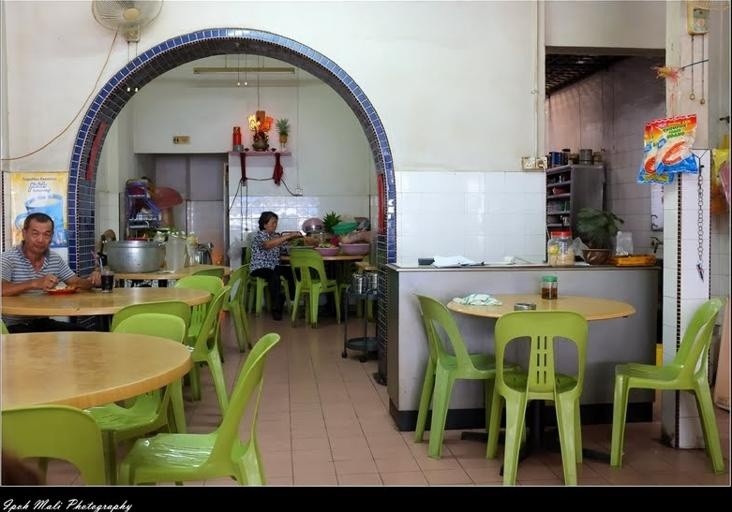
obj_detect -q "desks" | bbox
[387,261,659,433]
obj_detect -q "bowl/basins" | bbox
[339,243,369,255]
[314,247,339,256]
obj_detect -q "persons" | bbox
[0,212,102,333]
[249,211,302,321]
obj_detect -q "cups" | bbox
[100,271,114,293]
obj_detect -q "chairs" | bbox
[603,296,726,474]
[482,310,585,486]
[411,289,522,459]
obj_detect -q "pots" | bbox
[103,241,165,272]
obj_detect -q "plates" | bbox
[48,288,81,295]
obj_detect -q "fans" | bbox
[92,1,164,43]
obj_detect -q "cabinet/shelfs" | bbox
[546,164,607,235]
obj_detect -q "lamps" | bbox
[192,55,295,77]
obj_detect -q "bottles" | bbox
[616,231,634,256]
[548,232,575,265]
[541,275,558,300]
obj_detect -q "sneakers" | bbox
[273,312,282,320]
